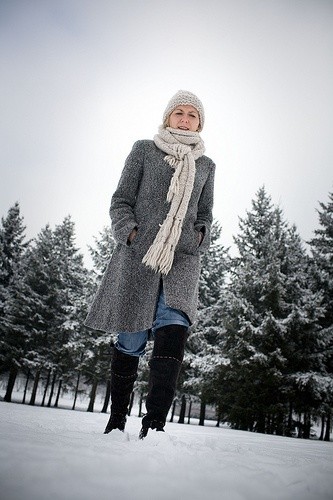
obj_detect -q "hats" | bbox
[163,89,205,133]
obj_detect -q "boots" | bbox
[140,336,185,439]
[105,347,140,433]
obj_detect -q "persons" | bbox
[82,90,217,442]
